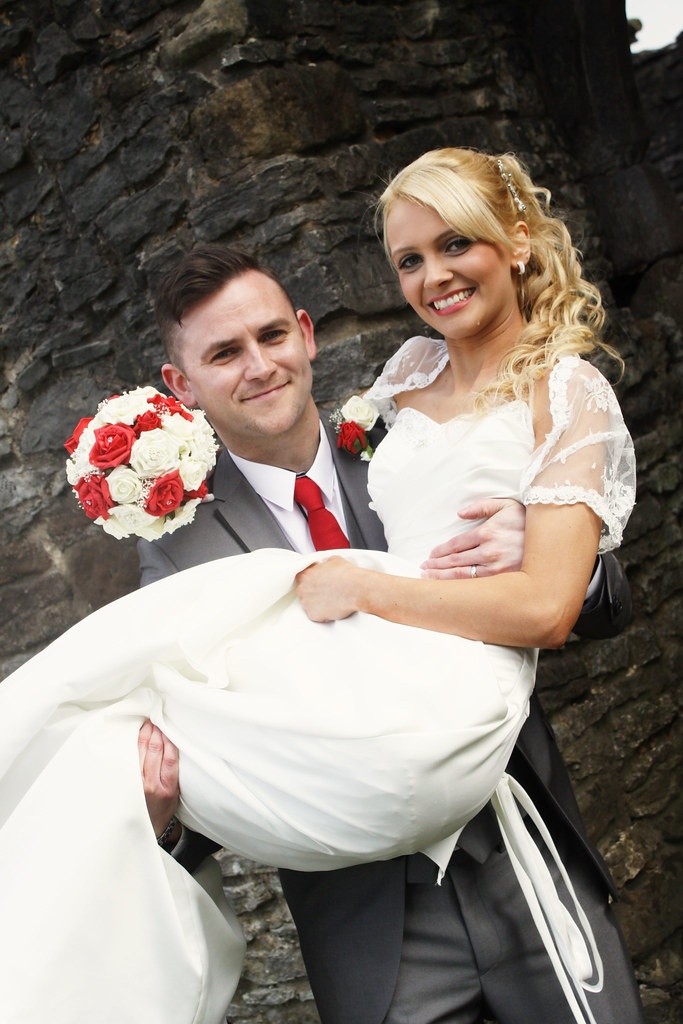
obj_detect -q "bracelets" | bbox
[157,818,179,846]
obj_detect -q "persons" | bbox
[139,246,642,1024]
[0,147,638,1024]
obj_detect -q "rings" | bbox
[470,567,477,578]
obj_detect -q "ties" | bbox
[288,475,355,551]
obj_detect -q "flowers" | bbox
[329,391,380,461]
[63,385,221,542]
